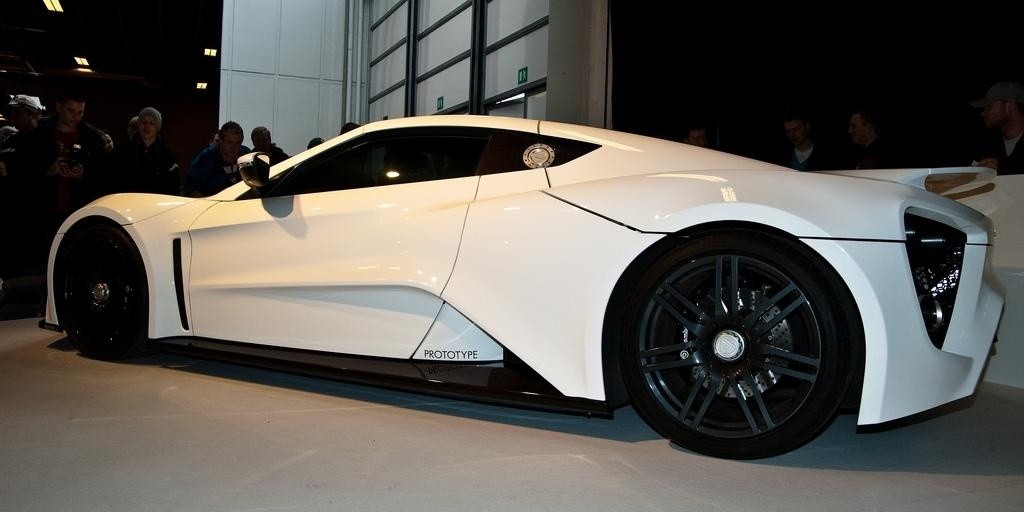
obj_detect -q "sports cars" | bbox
[38,113,1006,461]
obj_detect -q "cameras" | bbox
[68,144,92,175]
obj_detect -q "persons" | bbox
[681,126,712,149]
[838,109,900,173]
[338,121,358,136]
[774,110,826,174]
[249,126,291,168]
[187,121,253,200]
[1,90,182,314]
[954,80,1024,176]
[306,137,325,150]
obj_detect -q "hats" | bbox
[136,107,162,132]
